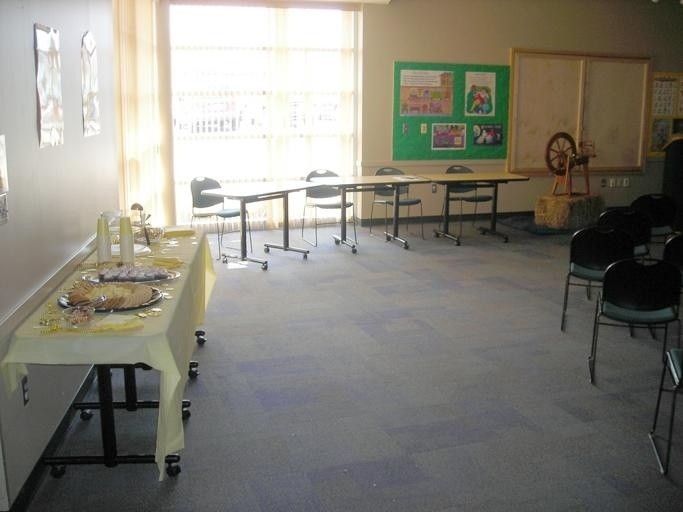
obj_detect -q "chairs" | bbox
[368,166,425,240]
[560,188,680,476]
[301,167,358,247]
[191,176,254,253]
[434,163,494,235]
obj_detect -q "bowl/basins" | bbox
[63,305,95,326]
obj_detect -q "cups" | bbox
[146,227,165,243]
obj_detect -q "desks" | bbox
[7,222,219,480]
[307,171,428,255]
[419,171,530,245]
[198,181,323,270]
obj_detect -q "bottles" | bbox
[118,209,133,263]
[97,210,111,264]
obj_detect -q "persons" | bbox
[469,89,487,112]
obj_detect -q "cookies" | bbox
[66,283,153,309]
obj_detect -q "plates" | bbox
[110,243,151,257]
